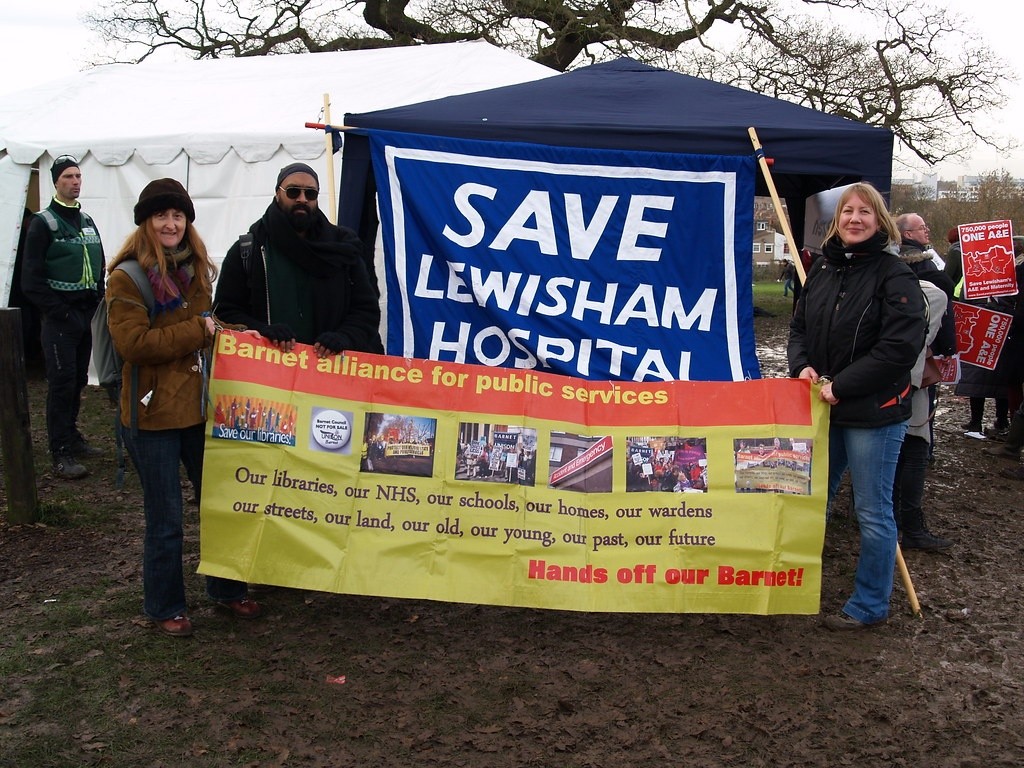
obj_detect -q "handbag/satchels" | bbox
[91,259,154,400]
[909,345,942,427]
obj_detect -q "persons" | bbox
[18,159,106,478]
[779,259,794,296]
[885,213,955,552]
[105,178,265,637]
[214,163,381,592]
[942,227,1024,481]
[786,185,926,631]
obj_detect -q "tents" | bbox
[337,59,894,239]
[0,40,563,385]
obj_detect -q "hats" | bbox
[948,228,959,243]
[134,178,195,225]
[276,163,320,194]
[52,155,80,185]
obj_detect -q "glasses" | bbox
[54,155,79,164]
[278,185,319,201]
[904,225,928,231]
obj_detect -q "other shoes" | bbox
[961,421,982,431]
[994,420,1005,428]
[999,465,1024,480]
[981,445,1020,460]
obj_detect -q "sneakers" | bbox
[50,448,88,479]
[825,612,884,632]
[72,433,106,457]
[217,596,261,618]
[898,529,955,551]
[152,617,192,636]
